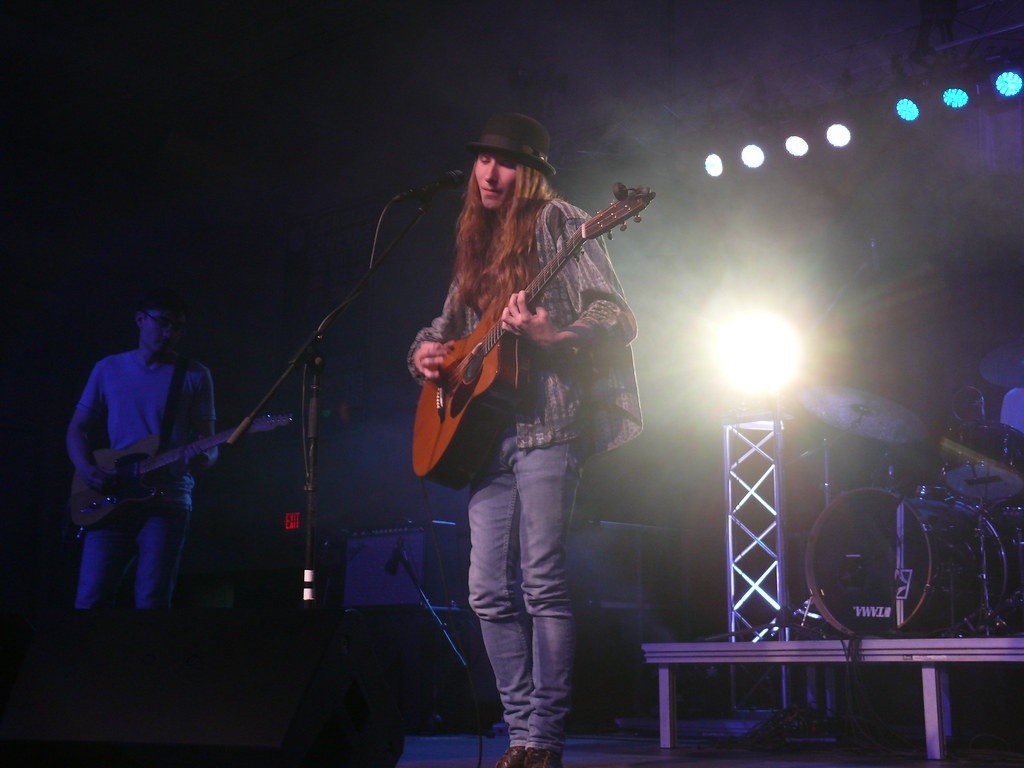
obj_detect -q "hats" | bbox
[465,112,556,177]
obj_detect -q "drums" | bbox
[803,485,1009,644]
[938,413,1024,505]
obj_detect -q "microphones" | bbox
[385,538,403,576]
[977,394,985,421]
[392,170,464,203]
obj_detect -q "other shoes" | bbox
[495,746,562,768]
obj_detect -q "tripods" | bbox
[942,491,1019,639]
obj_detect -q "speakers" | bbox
[0,605,406,768]
[345,521,458,607]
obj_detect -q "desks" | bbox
[641,636,1023,761]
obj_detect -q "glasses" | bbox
[142,310,188,337]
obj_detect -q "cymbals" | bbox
[803,385,931,445]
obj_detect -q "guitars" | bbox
[68,408,297,537]
[411,177,661,495]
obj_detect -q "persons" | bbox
[405,109,644,768]
[64,284,219,610]
